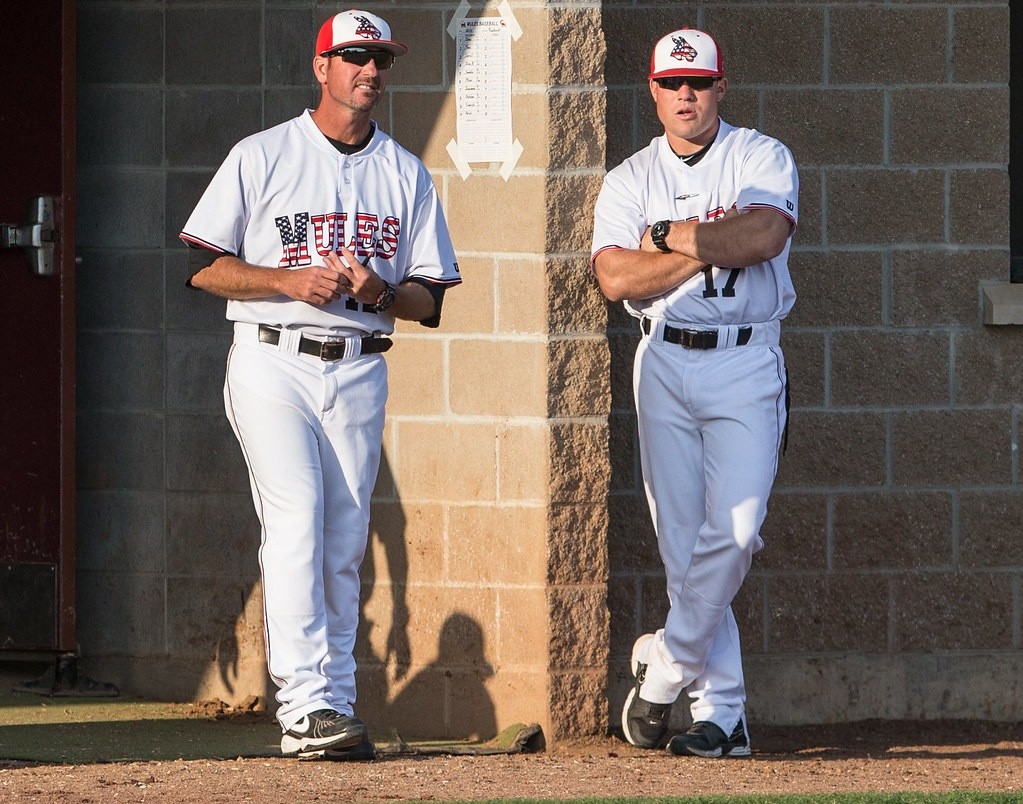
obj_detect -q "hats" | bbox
[314,9,408,57]
[648,27,725,78]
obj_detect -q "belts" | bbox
[644,317,753,349]
[258,327,393,362]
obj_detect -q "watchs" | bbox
[373,279,396,312]
[651,220,670,251]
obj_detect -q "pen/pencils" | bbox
[362,253,372,266]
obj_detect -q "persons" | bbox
[178,9,463,761]
[589,26,800,759]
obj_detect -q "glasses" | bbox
[321,46,394,70]
[653,76,722,91]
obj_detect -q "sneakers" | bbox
[666,711,752,757]
[298,735,376,762]
[280,708,367,757]
[621,634,673,748]
